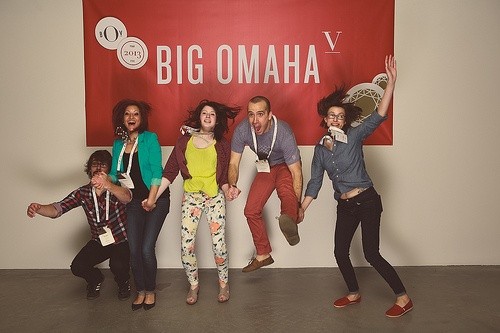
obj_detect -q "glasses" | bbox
[91,160,109,168]
[326,113,346,120]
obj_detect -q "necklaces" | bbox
[114,127,137,145]
[269,119,271,130]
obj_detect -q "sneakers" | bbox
[87,271,103,299]
[119,280,130,300]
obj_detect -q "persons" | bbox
[301,55,415,319]
[227,95,304,273]
[90,98,171,312]
[141,99,242,305]
[27,150,133,301]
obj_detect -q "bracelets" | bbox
[298,201,302,208]
[229,184,237,188]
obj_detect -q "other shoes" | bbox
[242,255,274,272]
[143,292,156,310]
[384,298,413,317]
[333,295,361,308]
[279,215,300,246]
[132,294,145,311]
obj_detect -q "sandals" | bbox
[218,283,230,303]
[186,284,200,304]
